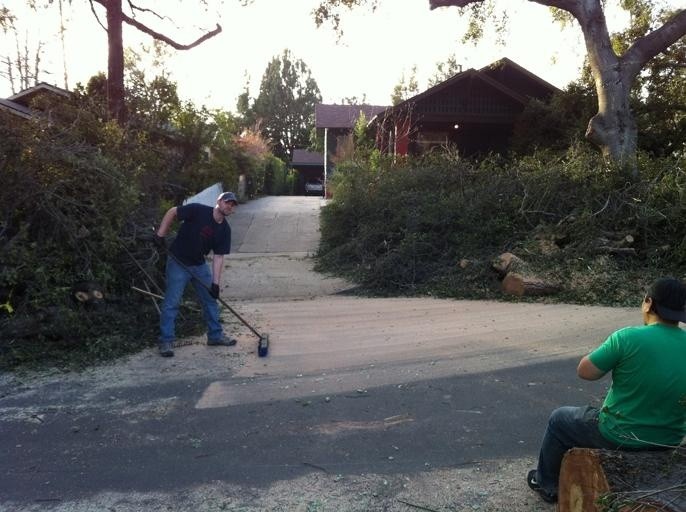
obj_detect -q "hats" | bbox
[649,278,686,323]
[217,191,237,206]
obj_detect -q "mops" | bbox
[152,238,269,357]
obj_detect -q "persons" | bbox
[526,276,686,504]
[155,192,236,357]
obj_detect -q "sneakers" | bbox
[159,342,174,357]
[528,469,558,503]
[207,336,236,346]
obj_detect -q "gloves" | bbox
[154,235,166,252]
[209,283,219,300]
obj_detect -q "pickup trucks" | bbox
[305,175,324,196]
